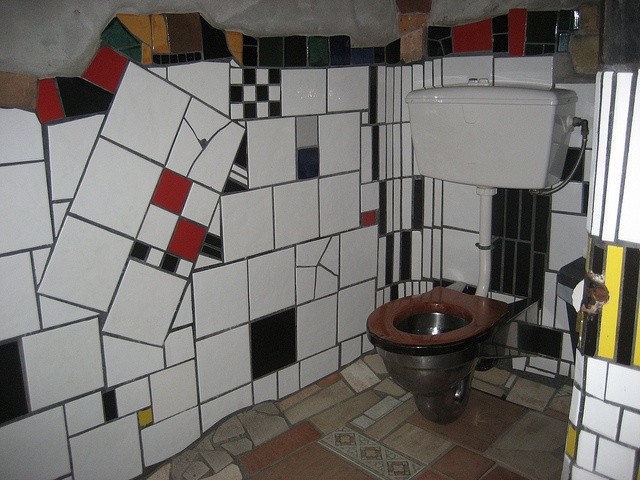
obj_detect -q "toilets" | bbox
[366,79,589,424]
